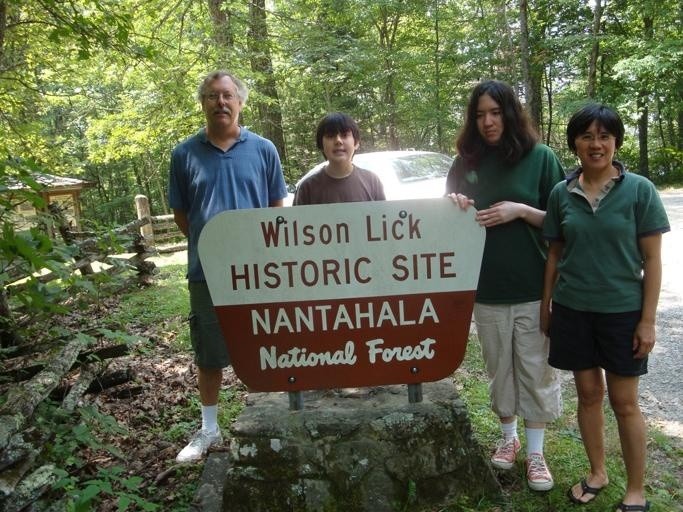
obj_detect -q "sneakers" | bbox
[491,437,521,469]
[175,424,223,464]
[525,452,554,491]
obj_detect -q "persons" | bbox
[292,111,386,205]
[443,80,568,492]
[167,70,287,464]
[540,103,671,512]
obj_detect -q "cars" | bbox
[276,149,458,211]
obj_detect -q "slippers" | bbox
[568,479,602,505]
[616,498,649,512]
